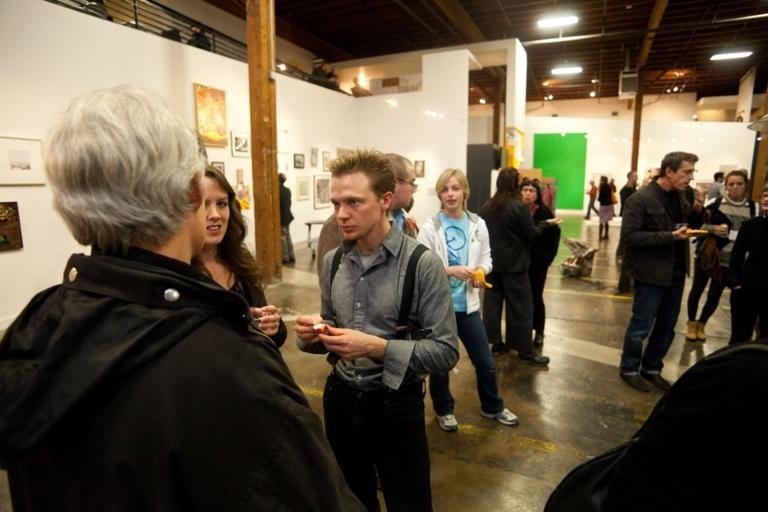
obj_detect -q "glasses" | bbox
[398,177,418,190]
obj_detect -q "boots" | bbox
[697,321,706,340]
[686,320,697,340]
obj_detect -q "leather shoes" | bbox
[639,369,671,391]
[619,372,650,392]
[492,342,509,356]
[519,355,550,367]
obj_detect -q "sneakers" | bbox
[436,413,458,432]
[533,333,544,348]
[480,406,519,426]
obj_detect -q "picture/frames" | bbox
[0,136,47,187]
[193,82,229,147]
[211,162,225,176]
[230,131,250,158]
[0,201,23,251]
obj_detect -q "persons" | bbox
[620,172,638,216]
[309,63,338,85]
[609,178,616,192]
[315,153,418,274]
[707,172,725,199]
[599,176,613,240]
[202,166,289,351]
[4,88,365,510]
[278,174,296,263]
[583,181,599,219]
[187,24,212,51]
[295,150,461,511]
[687,172,760,339]
[482,167,564,368]
[520,179,561,348]
[418,168,522,432]
[329,67,340,88]
[726,182,768,346]
[351,76,369,96]
[620,155,709,392]
